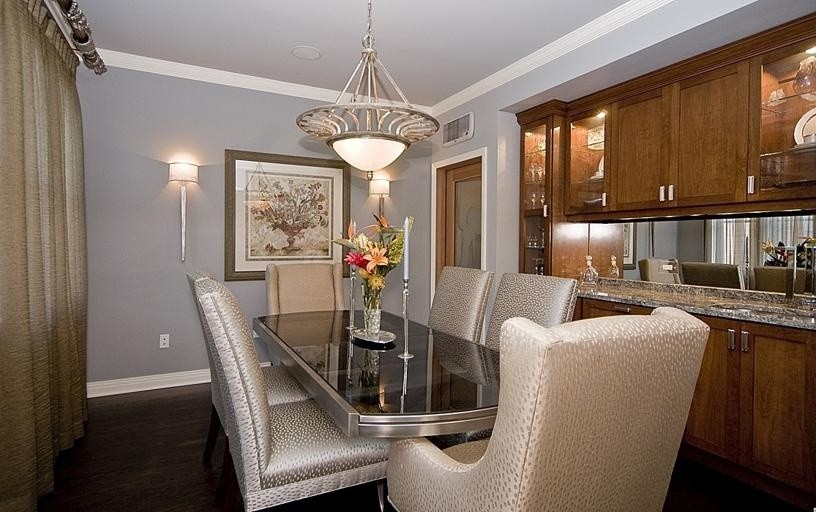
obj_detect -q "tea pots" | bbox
[791,55,816,103]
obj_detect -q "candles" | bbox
[403,215,409,279]
[352,220,356,267]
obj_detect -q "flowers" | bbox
[330,208,414,309]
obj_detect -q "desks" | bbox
[253,306,502,439]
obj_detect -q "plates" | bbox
[792,106,816,146]
[776,178,816,189]
[590,156,605,179]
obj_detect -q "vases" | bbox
[363,309,381,339]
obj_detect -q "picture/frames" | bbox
[224,148,351,281]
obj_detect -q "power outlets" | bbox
[159,333,169,349]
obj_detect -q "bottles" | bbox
[606,255,619,279]
[532,257,543,275]
[578,255,599,292]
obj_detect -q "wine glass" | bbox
[524,136,545,209]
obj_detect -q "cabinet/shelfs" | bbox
[515,11,816,512]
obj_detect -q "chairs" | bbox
[486,273,580,352]
[426,266,495,342]
[184,272,312,462]
[387,306,710,512]
[264,263,345,315]
[194,276,388,512]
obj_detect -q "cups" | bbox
[525,228,544,248]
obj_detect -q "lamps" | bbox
[296,1,440,181]
[169,162,199,262]
[369,180,390,219]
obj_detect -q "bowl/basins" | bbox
[582,198,602,208]
